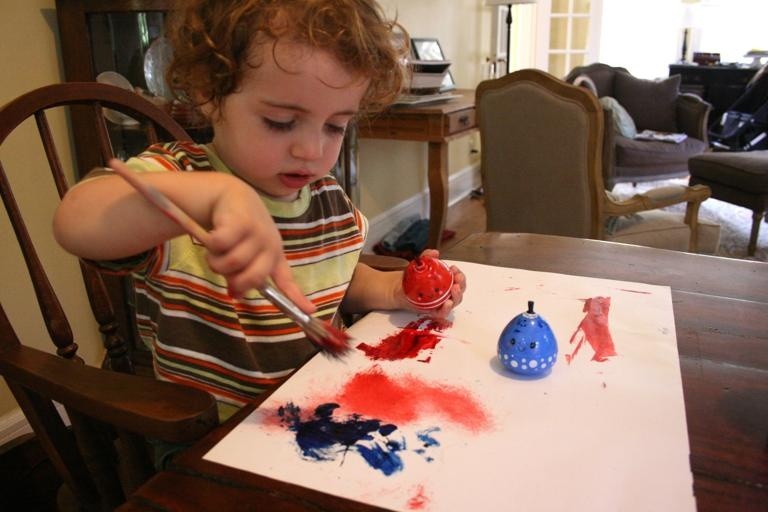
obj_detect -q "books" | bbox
[635,130,688,144]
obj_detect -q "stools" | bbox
[684,151,768,256]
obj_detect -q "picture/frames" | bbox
[410,38,455,94]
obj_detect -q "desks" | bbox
[110,231,767,512]
[344,89,478,249]
[670,64,765,131]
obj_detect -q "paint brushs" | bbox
[109,157,357,362]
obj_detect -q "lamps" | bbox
[485,0,536,76]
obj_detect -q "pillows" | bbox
[599,96,637,139]
[613,69,681,133]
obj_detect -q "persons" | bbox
[53,0,463,473]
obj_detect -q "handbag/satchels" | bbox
[706,110,763,152]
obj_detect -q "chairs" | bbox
[0,81,410,511]
[564,63,715,192]
[476,69,721,255]
